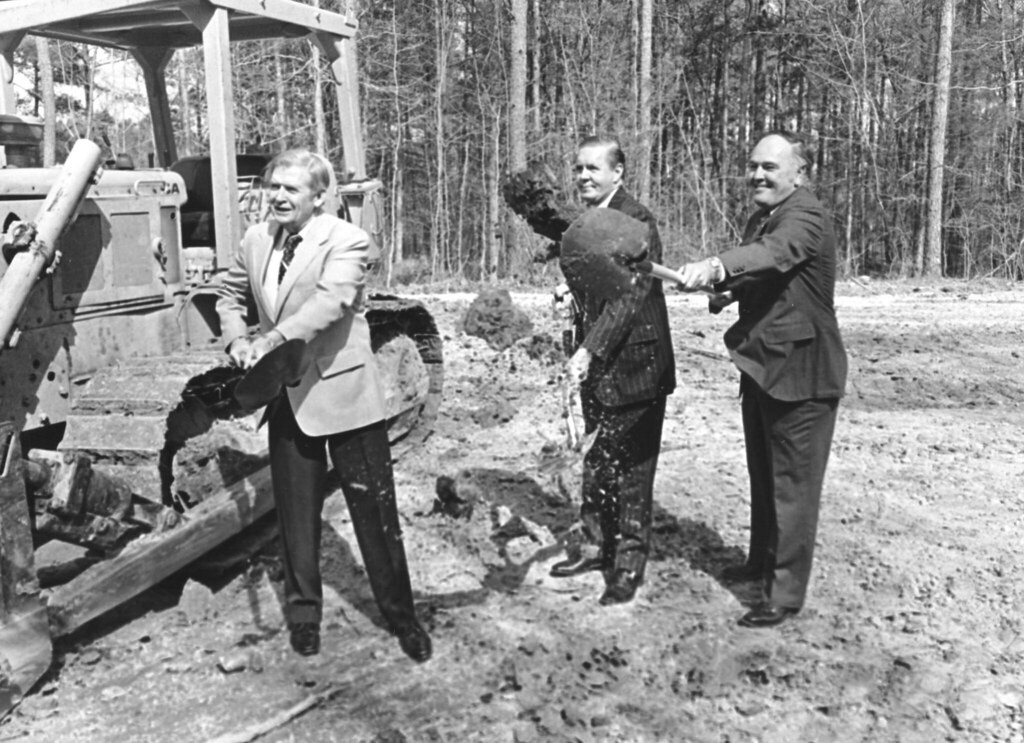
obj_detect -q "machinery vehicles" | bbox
[2,0,448,724]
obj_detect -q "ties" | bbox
[278,235,303,286]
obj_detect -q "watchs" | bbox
[707,257,722,283]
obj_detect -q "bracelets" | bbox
[261,332,278,348]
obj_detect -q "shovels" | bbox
[235,340,308,408]
[560,209,705,300]
[548,384,598,483]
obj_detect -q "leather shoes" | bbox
[400,620,431,662]
[551,553,611,575]
[722,566,775,584]
[599,567,644,605]
[741,601,790,627]
[290,622,320,656]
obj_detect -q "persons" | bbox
[548,134,680,607]
[213,145,433,666]
[674,128,854,630]
